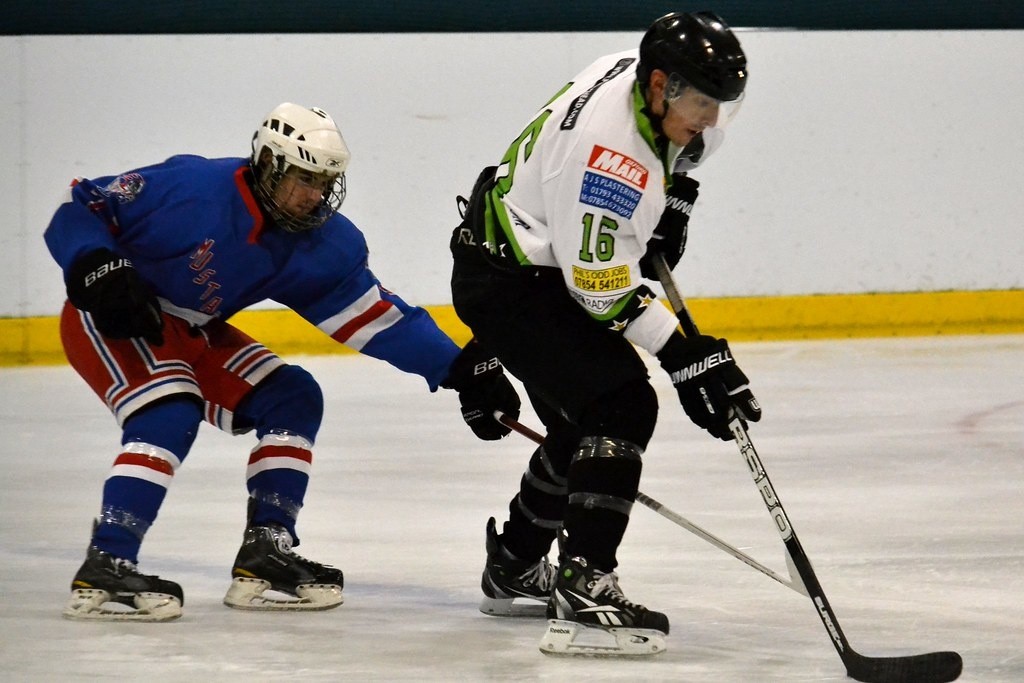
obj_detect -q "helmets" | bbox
[639,10,748,119]
[251,102,351,233]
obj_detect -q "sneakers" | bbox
[540,527,669,659]
[62,519,185,622]
[479,517,556,617]
[222,497,343,611]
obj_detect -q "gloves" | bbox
[441,350,520,441]
[640,173,699,282]
[66,247,167,347]
[656,334,760,441]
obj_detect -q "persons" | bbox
[41,101,522,622]
[448,5,764,660]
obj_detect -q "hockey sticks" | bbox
[653,251,962,683]
[494,410,811,599]
